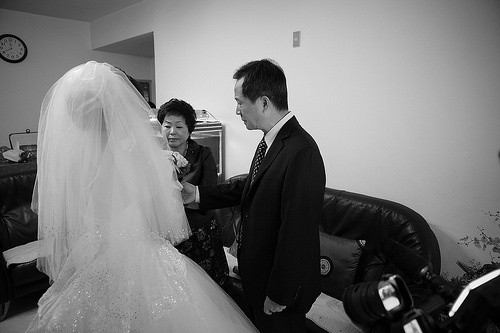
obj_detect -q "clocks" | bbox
[0,34,27,63]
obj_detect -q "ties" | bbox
[249,137,267,188]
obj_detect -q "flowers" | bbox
[172,151,191,176]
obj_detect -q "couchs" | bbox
[218,175,441,333]
[0,160,49,321]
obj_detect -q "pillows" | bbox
[319,231,366,299]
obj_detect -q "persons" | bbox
[157,98,230,288]
[179,59,326,333]
[25,60,260,333]
